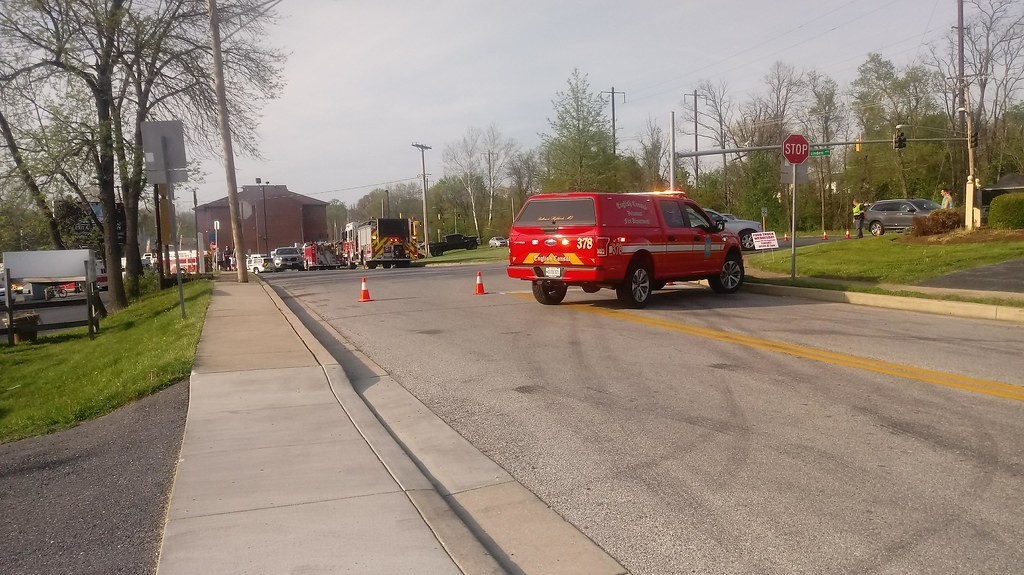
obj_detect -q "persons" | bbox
[233,248,237,271]
[941,188,955,210]
[223,245,233,271]
[853,199,873,239]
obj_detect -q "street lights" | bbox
[956,81,975,181]
[256,178,269,256]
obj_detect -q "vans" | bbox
[92,249,276,289]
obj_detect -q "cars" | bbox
[686,208,763,251]
[489,237,507,248]
[506,239,509,247]
[0,288,16,305]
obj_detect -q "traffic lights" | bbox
[971,132,980,148]
[894,131,907,149]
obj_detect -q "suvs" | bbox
[505,191,744,310]
[272,247,306,272]
[861,198,949,234]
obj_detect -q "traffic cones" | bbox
[355,276,375,302]
[845,227,851,239]
[822,230,829,240]
[473,272,489,295]
[783,232,789,242]
[876,228,882,238]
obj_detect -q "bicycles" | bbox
[47,286,68,299]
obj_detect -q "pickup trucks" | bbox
[428,234,481,257]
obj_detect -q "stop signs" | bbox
[210,242,216,251]
[783,134,809,165]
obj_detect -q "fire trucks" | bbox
[342,216,424,269]
